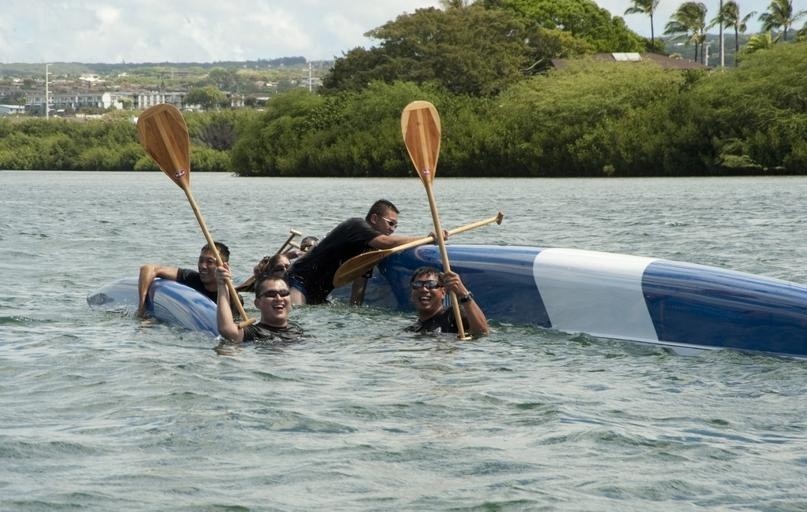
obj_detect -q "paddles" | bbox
[240,229,302,288]
[137,104,257,329]
[401,100,472,341]
[333,212,504,288]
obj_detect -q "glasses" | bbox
[378,214,397,228]
[411,280,443,289]
[258,290,290,298]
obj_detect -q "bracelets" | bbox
[428,232,438,246]
[459,291,473,303]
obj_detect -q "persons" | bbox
[404,266,489,340]
[285,198,449,306]
[137,242,244,316]
[296,236,333,303]
[268,254,291,278]
[214,250,303,345]
